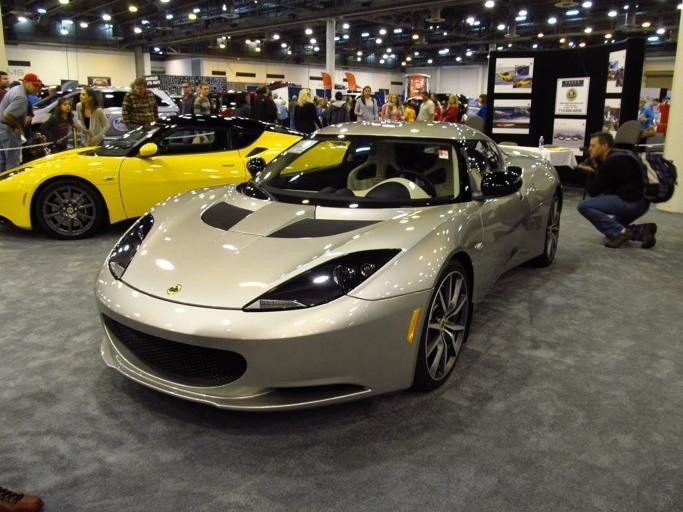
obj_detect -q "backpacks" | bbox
[608,147,678,203]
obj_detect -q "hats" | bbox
[23,74,41,85]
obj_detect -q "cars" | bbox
[333,91,465,114]
[551,131,582,142]
[495,70,514,82]
[512,78,530,88]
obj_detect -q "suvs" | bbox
[24,84,182,143]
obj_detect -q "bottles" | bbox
[539,136,544,150]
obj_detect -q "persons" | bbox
[638,95,670,153]
[1,486,44,512]
[576,131,657,249]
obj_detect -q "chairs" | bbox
[415,145,469,200]
[343,141,406,193]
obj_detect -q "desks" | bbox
[535,144,589,201]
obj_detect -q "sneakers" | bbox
[605,229,633,246]
[640,223,656,248]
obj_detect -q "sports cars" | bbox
[92,116,565,413]
[0,109,351,244]
